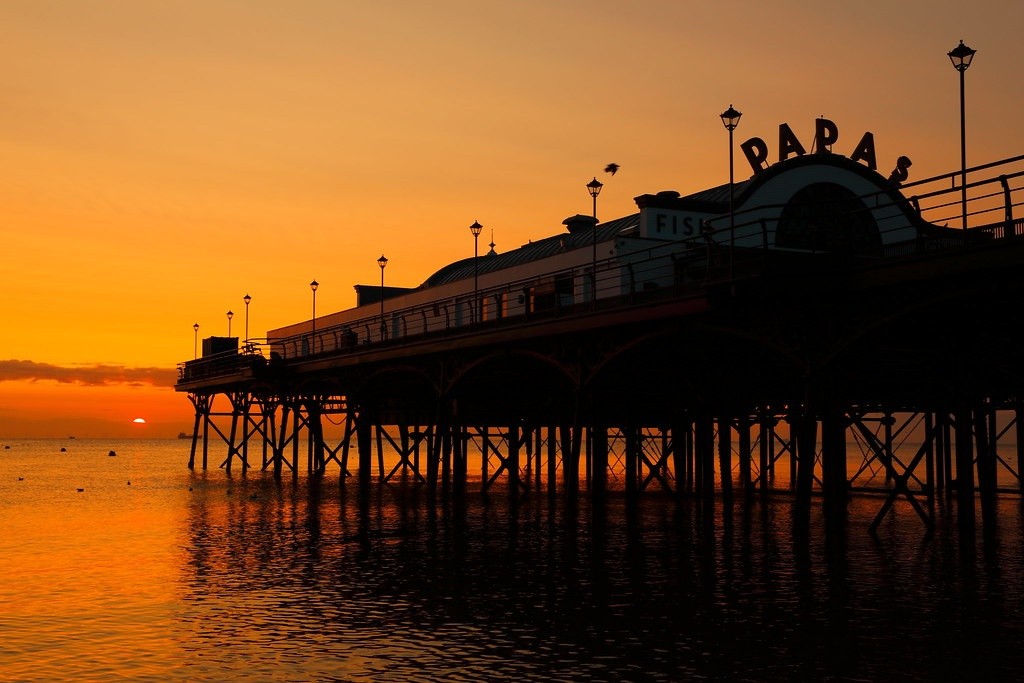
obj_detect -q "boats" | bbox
[178,432,203,439]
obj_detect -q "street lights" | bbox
[310,279,319,331]
[377,254,388,314]
[720,105,742,248]
[948,40,977,230]
[244,294,251,340]
[193,324,199,360]
[586,176,604,301]
[227,311,233,338]
[470,220,483,324]
